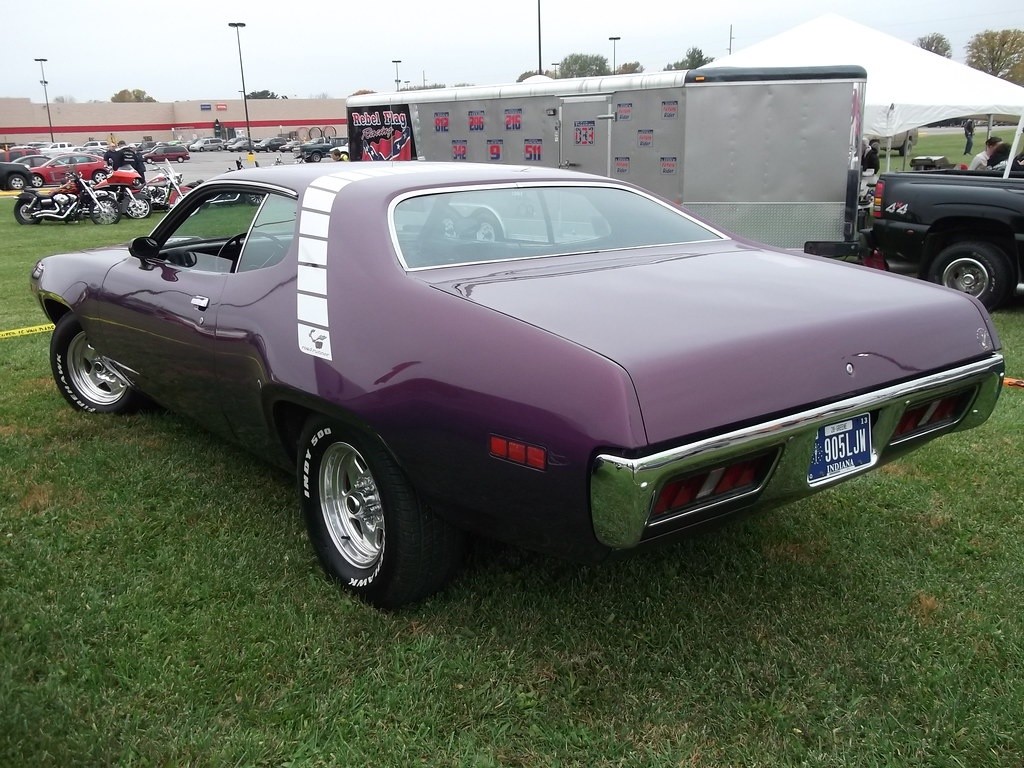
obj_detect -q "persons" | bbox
[861,136,881,198]
[962,118,976,156]
[967,136,1024,171]
[104,140,146,186]
[331,149,349,162]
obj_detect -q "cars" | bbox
[223,137,255,153]
[116,142,189,164]
[30,162,1008,616]
[0,139,115,191]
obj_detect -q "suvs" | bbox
[255,136,351,162]
[169,138,227,152]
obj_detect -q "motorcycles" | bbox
[13,152,308,225]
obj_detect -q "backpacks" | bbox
[120,149,136,166]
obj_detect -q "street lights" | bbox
[552,64,560,81]
[392,60,401,91]
[35,58,54,142]
[609,36,621,74]
[228,22,254,160]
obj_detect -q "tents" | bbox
[694,16,1024,180]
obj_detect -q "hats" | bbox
[128,144,136,147]
[117,140,126,144]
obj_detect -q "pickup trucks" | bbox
[861,171,1024,314]
